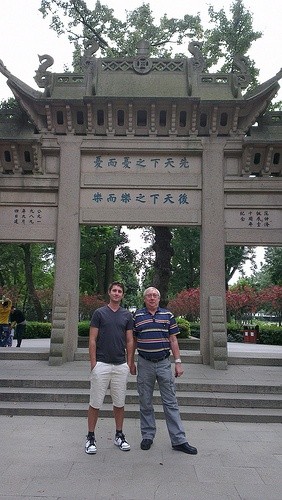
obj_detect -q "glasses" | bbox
[145,294,159,297]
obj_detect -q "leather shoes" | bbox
[172,443,197,454]
[141,439,153,450]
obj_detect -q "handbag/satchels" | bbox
[19,319,26,325]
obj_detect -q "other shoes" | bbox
[16,344,20,347]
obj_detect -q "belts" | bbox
[139,352,170,363]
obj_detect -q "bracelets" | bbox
[175,358,181,364]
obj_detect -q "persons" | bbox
[0,298,26,347]
[85,281,134,454]
[130,287,198,455]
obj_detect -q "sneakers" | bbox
[85,436,97,454]
[114,433,131,451]
